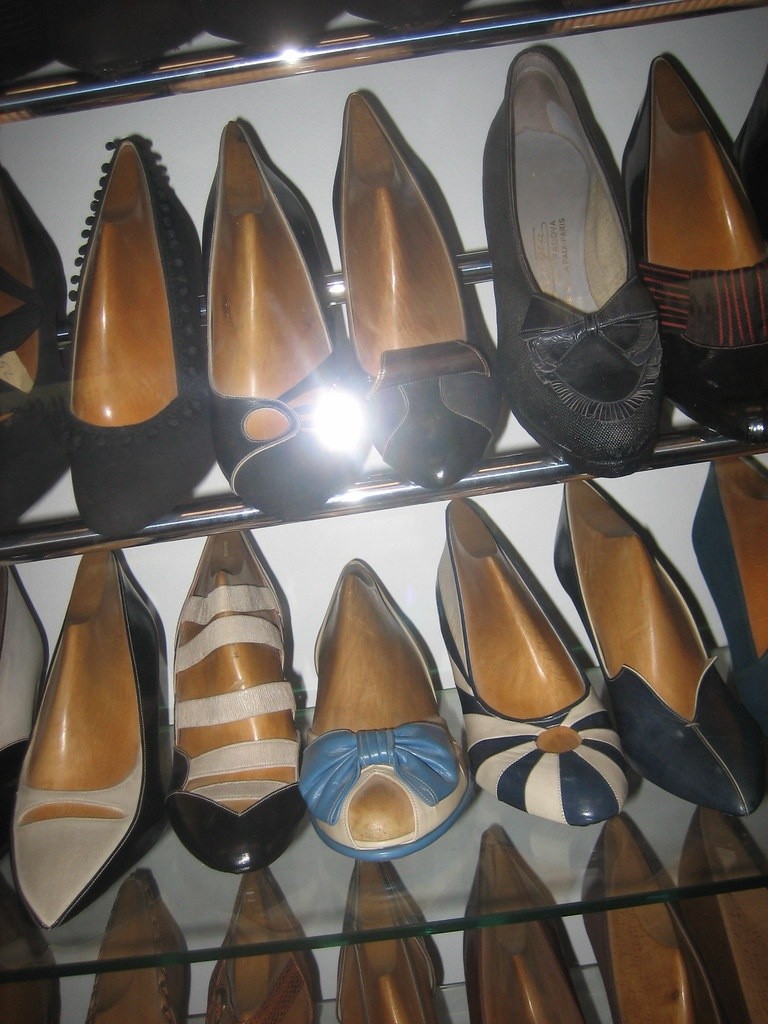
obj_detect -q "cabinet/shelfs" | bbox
[0,0,767,1024]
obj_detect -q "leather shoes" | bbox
[9,549,168,930]
[622,52,768,443]
[679,804,768,1024]
[203,119,370,514]
[205,868,320,1022]
[483,45,666,476]
[336,857,437,1024]
[165,530,304,875]
[691,455,767,735]
[554,479,767,816]
[331,91,508,486]
[0,563,47,775]
[85,870,191,1023]
[298,558,472,861]
[462,823,586,1024]
[436,498,628,827]
[0,161,71,523]
[580,811,722,1024]
[68,135,216,537]
